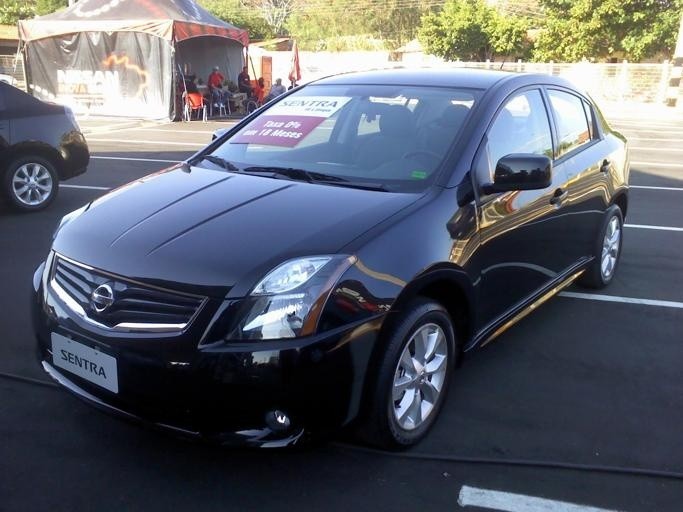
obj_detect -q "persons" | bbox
[288,80,298,91]
[262,78,286,105]
[186,76,203,109]
[238,66,256,98]
[242,77,266,113]
[207,65,231,108]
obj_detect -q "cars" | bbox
[0,80,90,213]
[30,66,629,449]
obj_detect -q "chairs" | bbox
[185,87,269,123]
[356,99,535,175]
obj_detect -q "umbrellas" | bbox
[287,39,302,87]
